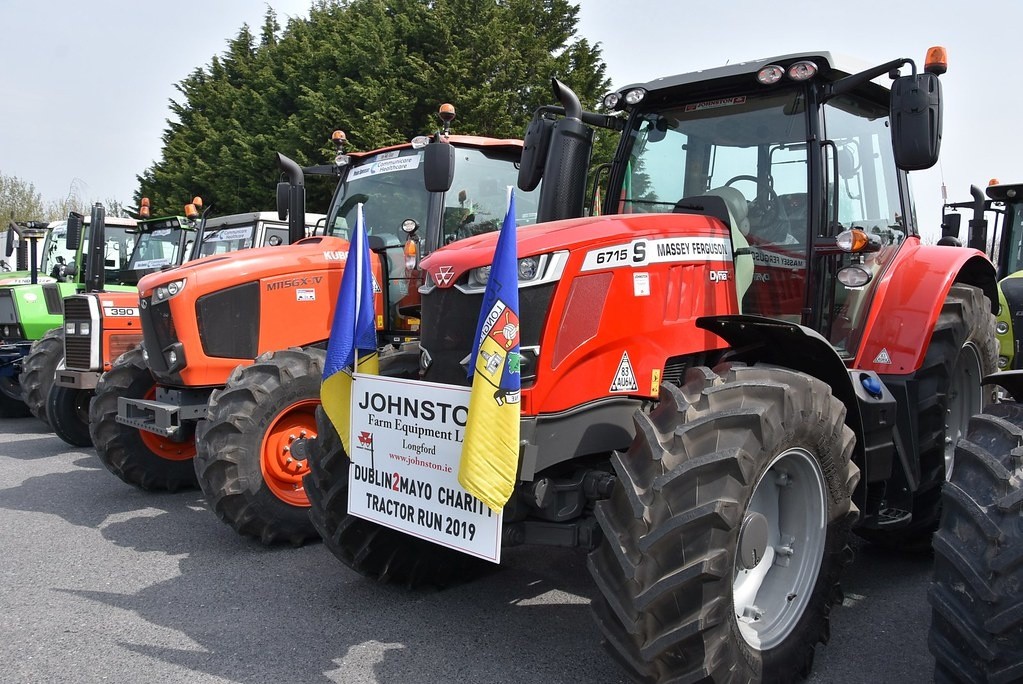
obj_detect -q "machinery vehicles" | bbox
[301,43,1023,684]
[0,204,348,448]
[87,100,583,550]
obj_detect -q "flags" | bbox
[320,206,379,460]
[457,188,521,515]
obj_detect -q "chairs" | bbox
[761,192,808,245]
